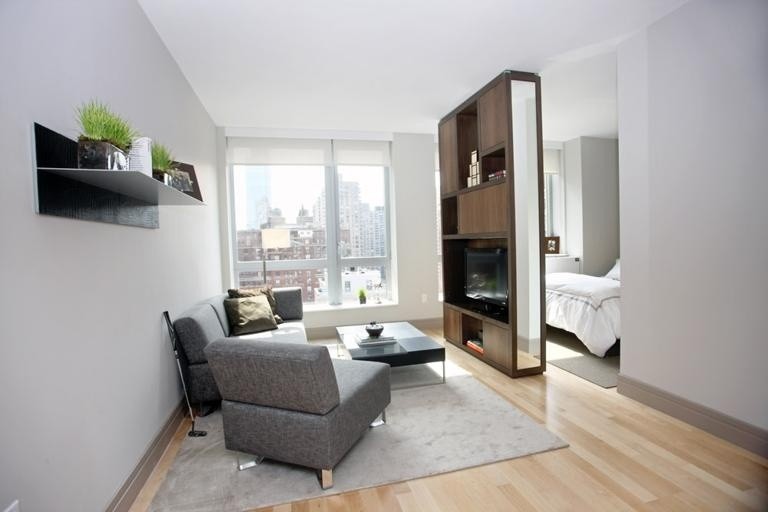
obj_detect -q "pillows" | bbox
[226,286,280,332]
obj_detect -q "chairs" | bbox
[201,338,392,490]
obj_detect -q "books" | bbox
[466,340,483,353]
[489,170,506,181]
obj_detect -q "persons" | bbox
[171,172,191,191]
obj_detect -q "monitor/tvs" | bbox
[463,246,508,316]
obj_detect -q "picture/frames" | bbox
[153,143,172,186]
[169,161,204,201]
[545,237,560,253]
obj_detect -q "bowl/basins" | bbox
[365,328,384,338]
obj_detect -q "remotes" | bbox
[358,338,397,346]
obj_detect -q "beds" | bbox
[545,256,621,357]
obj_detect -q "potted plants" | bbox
[358,289,367,304]
[68,97,130,172]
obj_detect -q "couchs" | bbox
[174,287,309,424]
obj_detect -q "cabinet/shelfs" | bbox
[435,69,545,378]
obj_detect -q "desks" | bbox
[334,319,447,384]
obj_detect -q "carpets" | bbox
[546,348,620,388]
[142,331,567,510]
